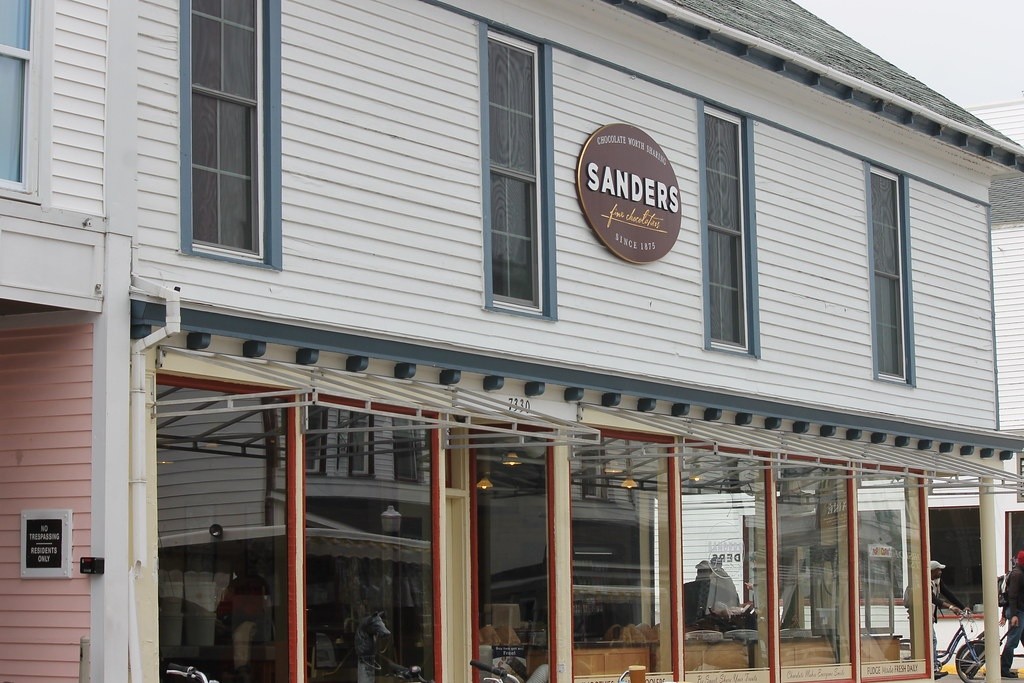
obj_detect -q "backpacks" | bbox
[904,585,911,608]
[998,571,1013,607]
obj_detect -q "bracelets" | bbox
[949,605,954,610]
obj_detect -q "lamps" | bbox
[477,478,493,489]
[690,474,704,480]
[621,480,638,489]
[503,454,521,466]
[381,506,401,531]
[604,467,622,473]
[210,523,223,538]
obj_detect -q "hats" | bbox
[931,560,946,571]
[1018,551,1024,567]
[695,560,712,570]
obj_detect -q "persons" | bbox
[684,560,713,625]
[997,551,1024,678]
[904,561,971,680]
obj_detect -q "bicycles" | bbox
[899,609,986,683]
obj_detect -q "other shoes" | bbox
[935,671,948,679]
[1001,670,1018,678]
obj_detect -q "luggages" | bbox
[960,623,1018,679]
